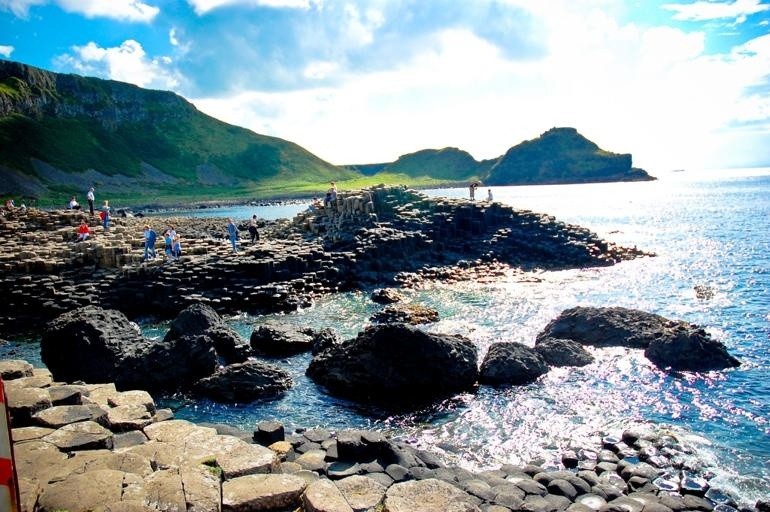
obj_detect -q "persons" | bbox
[74,219,90,242]
[324,182,338,207]
[227,218,238,253]
[86,187,96,215]
[69,196,82,210]
[174,234,181,260]
[102,200,110,230]
[326,187,337,209]
[165,234,174,263]
[142,225,158,261]
[164,224,179,256]
[248,215,261,242]
[486,189,494,201]
[469,182,476,201]
[308,197,321,213]
[15,201,28,213]
[5,199,16,211]
